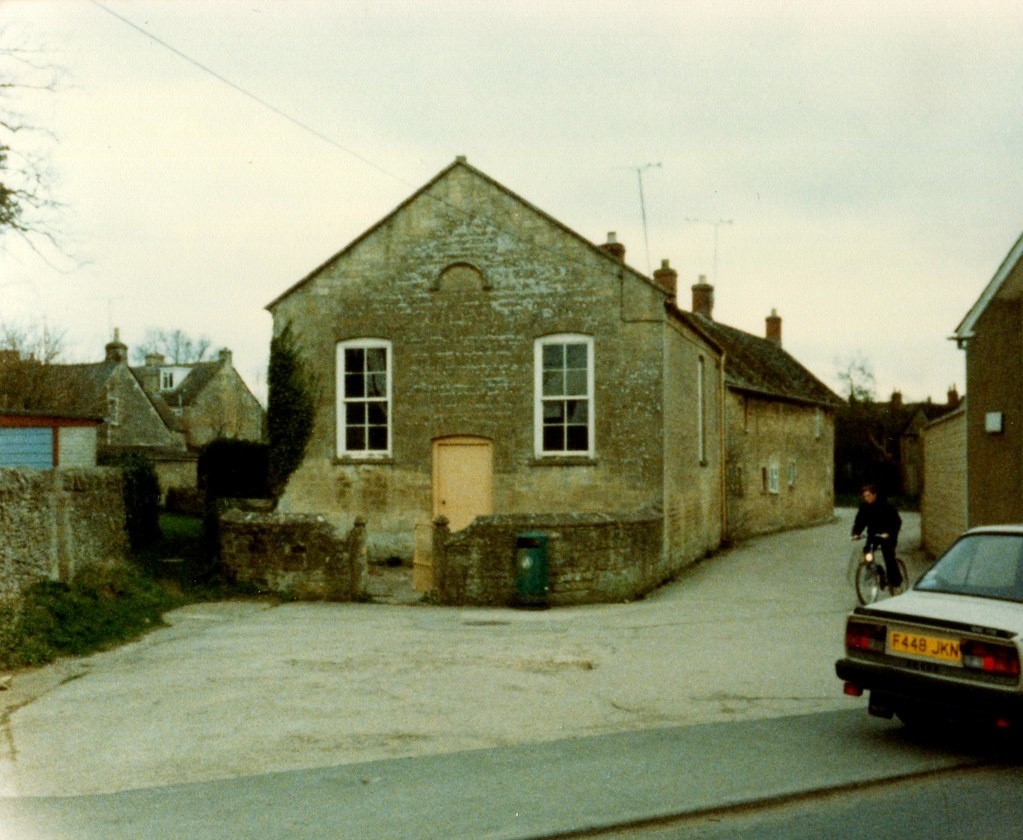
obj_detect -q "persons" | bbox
[851,484,904,588]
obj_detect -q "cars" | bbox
[835,524,1022,758]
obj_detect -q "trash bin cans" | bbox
[513,530,552,610]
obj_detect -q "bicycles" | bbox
[848,531,909,604]
